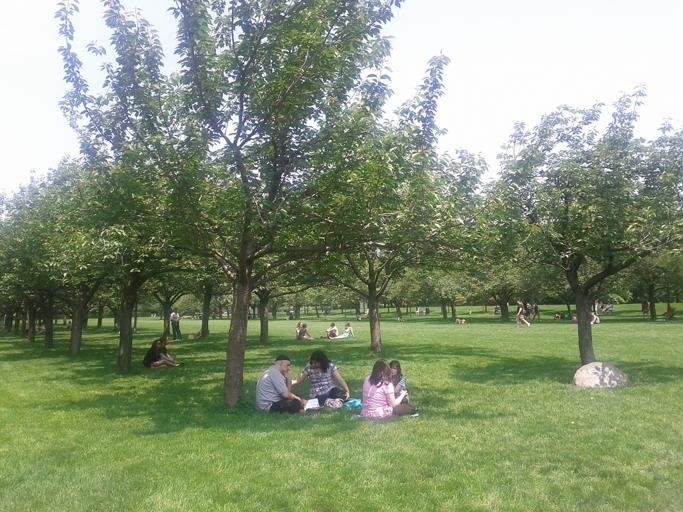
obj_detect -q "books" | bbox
[302,398,325,413]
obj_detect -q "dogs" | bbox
[455,318,465,329]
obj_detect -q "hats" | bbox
[276,355,290,360]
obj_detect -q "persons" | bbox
[298,323,314,341]
[327,323,356,340]
[358,358,418,420]
[141,333,184,370]
[389,360,409,405]
[522,299,542,324]
[589,311,600,325]
[169,307,184,344]
[291,351,350,409]
[641,298,651,320]
[253,354,307,415]
[514,297,531,329]
[295,321,303,337]
[325,322,338,339]
